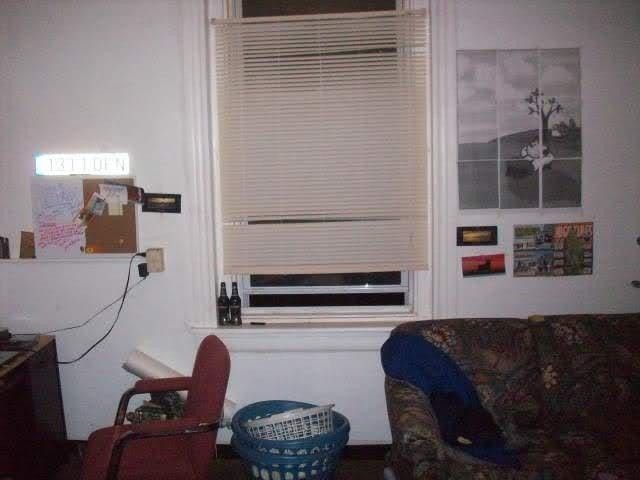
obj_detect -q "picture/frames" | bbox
[457,226,497,245]
[142,193,181,213]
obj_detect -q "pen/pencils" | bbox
[251,322,265,324]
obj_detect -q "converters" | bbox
[138,263,149,277]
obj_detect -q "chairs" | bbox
[80,335,230,480]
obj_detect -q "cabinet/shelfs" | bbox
[0,334,66,480]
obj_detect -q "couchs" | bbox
[385,313,640,480]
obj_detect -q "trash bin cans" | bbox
[230,399,350,480]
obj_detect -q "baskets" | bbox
[230,400,350,480]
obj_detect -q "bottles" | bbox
[229,281,243,327]
[217,281,231,325]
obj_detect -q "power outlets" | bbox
[146,248,163,271]
